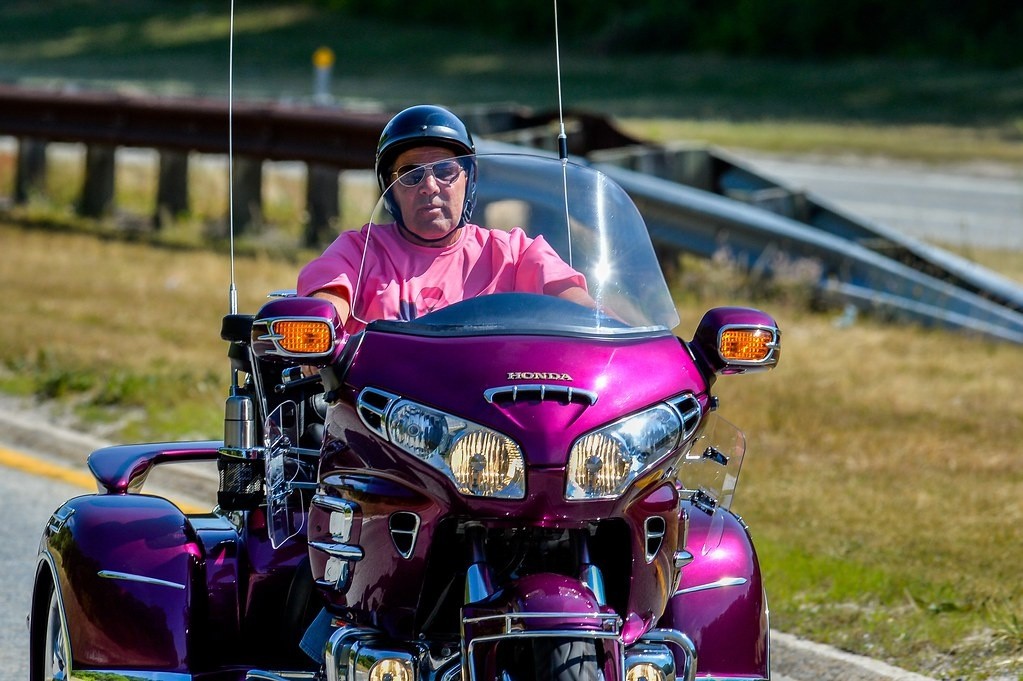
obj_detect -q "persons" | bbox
[297,104,632,379]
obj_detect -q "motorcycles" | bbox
[26,0,785,680]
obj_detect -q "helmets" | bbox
[374,104,478,241]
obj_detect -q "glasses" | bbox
[391,161,465,188]
